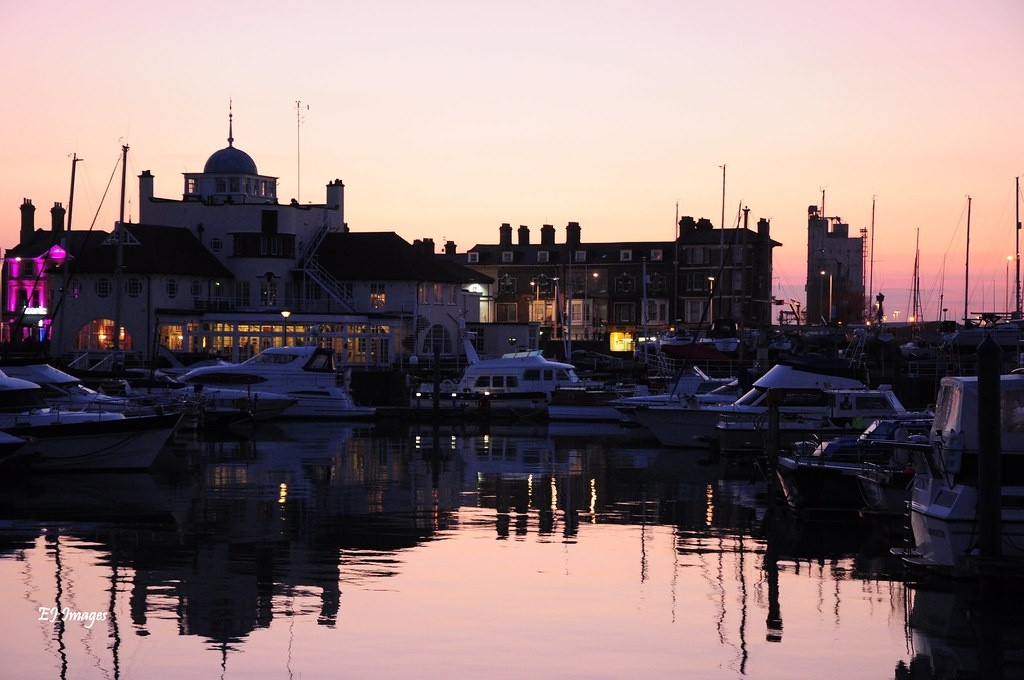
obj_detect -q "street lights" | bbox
[281,310,291,346]
[707,276,715,325]
[593,272,599,340]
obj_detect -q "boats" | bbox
[614,358,870,449]
[0,364,207,472]
[691,384,907,457]
[775,409,935,517]
[408,349,604,407]
[855,421,930,520]
[122,368,300,423]
[656,177,1023,378]
[887,374,1024,578]
[547,362,736,420]
[175,346,377,420]
[605,364,742,448]
